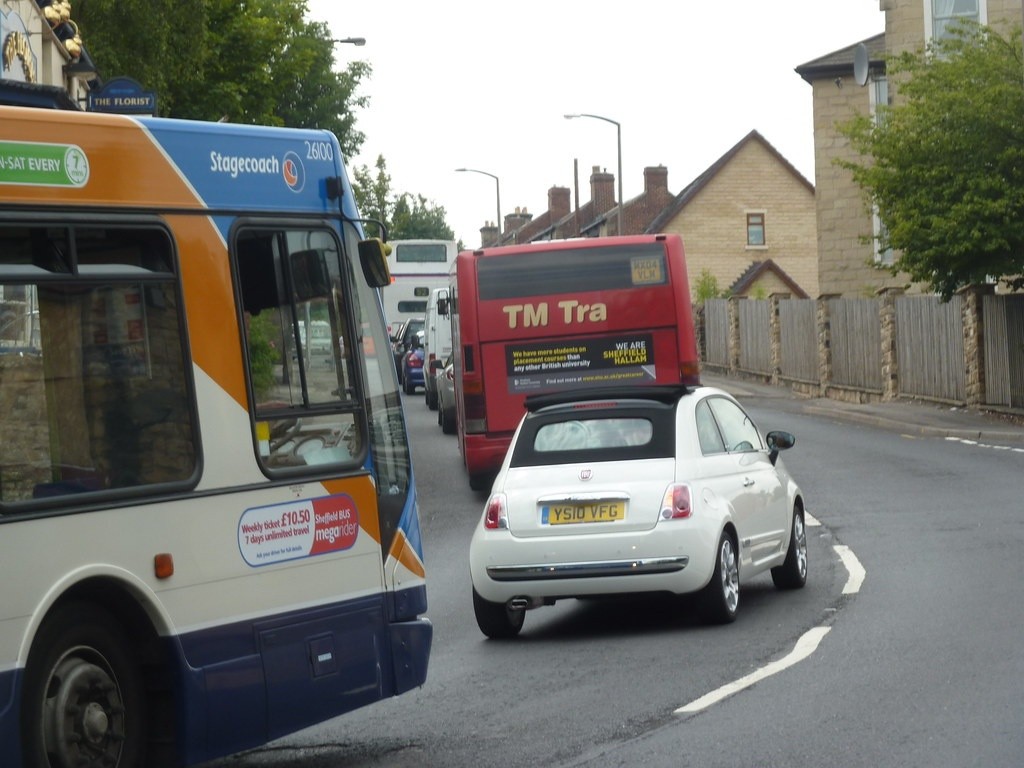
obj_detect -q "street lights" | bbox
[563,113,624,239]
[455,169,502,248]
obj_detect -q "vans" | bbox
[411,288,451,410]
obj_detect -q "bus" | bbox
[438,233,701,490]
[379,239,458,346]
[0,105,434,768]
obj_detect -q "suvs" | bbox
[390,318,426,385]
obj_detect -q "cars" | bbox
[431,350,456,434]
[339,322,376,359]
[469,383,808,637]
[289,320,338,362]
[401,331,426,395]
